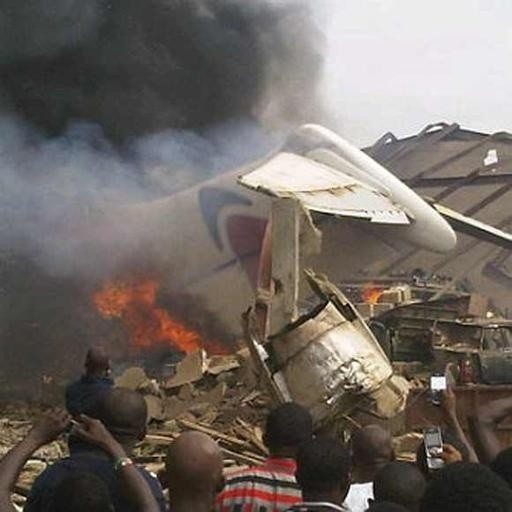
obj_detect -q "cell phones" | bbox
[67,418,79,437]
[423,424,446,470]
[431,369,449,405]
